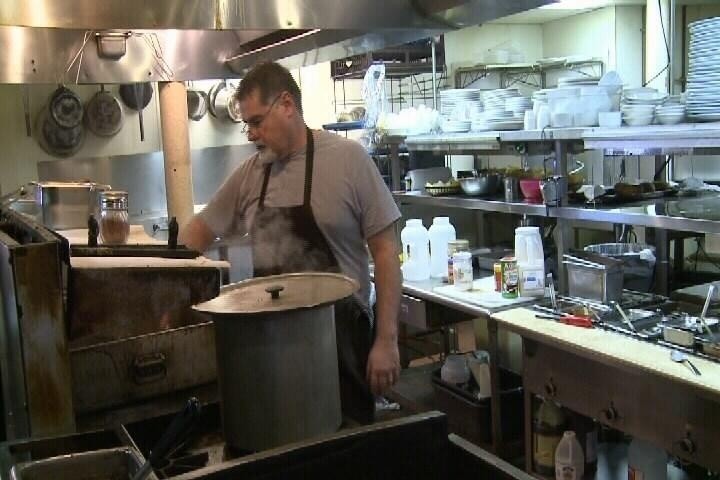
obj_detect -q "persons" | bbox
[178,63,403,396]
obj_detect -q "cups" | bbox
[523,102,552,130]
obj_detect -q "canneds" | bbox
[447,239,471,287]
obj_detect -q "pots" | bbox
[83,84,267,136]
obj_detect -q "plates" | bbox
[436,16,720,132]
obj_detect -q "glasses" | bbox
[242,94,282,135]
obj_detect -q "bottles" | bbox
[527,394,631,479]
[97,191,134,247]
[503,176,519,202]
[401,216,545,299]
[468,350,496,401]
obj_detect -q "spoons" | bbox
[667,349,703,378]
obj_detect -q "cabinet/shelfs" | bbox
[391,121,719,311]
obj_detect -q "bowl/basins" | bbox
[520,178,540,200]
[598,72,624,87]
[622,103,688,127]
[459,173,501,197]
